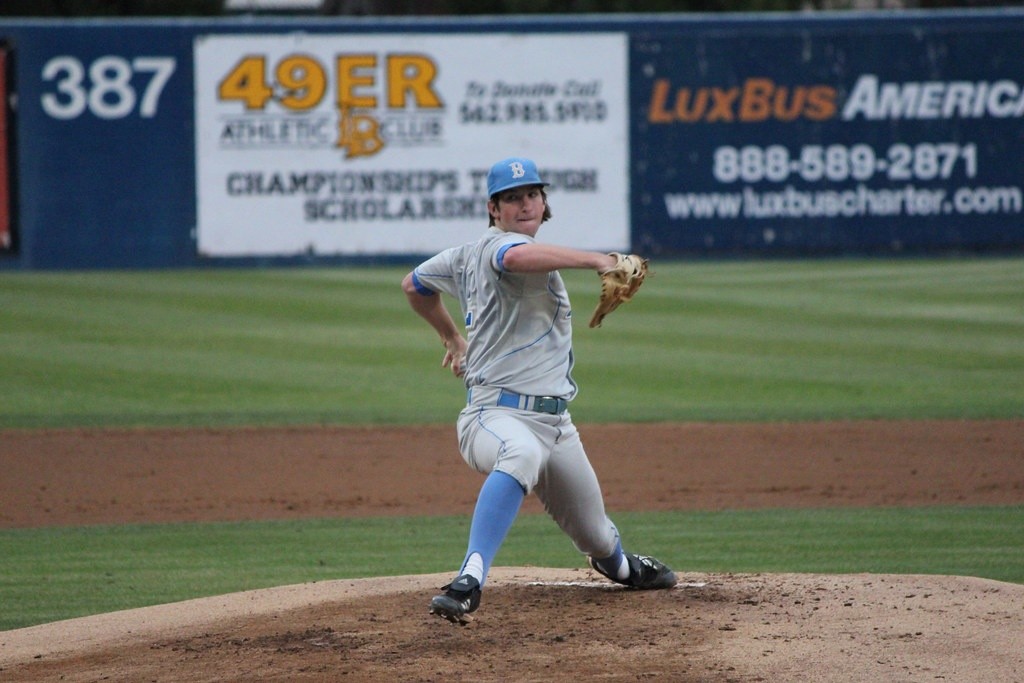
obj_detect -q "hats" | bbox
[486,157,550,203]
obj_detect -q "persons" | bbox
[401,158,679,628]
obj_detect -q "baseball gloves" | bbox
[589,250,649,331]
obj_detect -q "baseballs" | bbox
[450,356,467,378]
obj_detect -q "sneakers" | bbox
[429,574,481,626]
[586,554,678,590]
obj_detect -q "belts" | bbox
[498,393,567,414]
